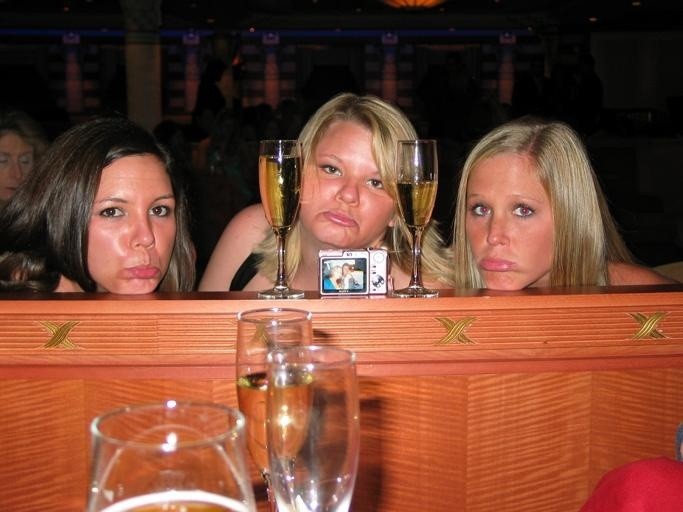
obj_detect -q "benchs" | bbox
[0,283,683,512]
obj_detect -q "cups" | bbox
[265,345,359,512]
[83,398,252,511]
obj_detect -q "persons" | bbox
[197,94,453,291]
[321,262,344,291]
[0,109,47,208]
[151,96,314,290]
[336,263,360,290]
[0,116,196,294]
[451,119,681,288]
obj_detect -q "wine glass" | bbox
[254,139,310,294]
[389,138,445,298]
[235,308,314,512]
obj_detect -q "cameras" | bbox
[319,246,390,296]
